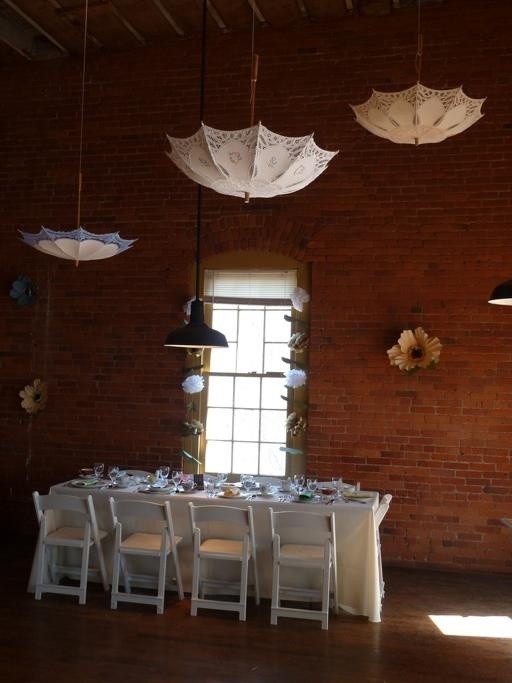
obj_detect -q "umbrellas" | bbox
[346,32,488,145]
[165,51,340,203]
[18,164,140,267]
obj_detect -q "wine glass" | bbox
[160,465,183,491]
[217,469,253,494]
[293,474,343,501]
[94,462,120,486]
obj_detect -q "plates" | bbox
[71,478,113,487]
[217,491,252,498]
[214,481,260,489]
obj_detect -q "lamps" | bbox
[161,0,228,349]
[488,279,512,307]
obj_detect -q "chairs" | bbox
[31,490,110,604]
[267,508,339,630]
[121,469,359,491]
[374,493,393,557]
[107,496,183,614]
[186,502,259,621]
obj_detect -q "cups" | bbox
[259,483,273,496]
[78,468,94,478]
[341,485,356,495]
[182,480,198,491]
[118,474,129,488]
